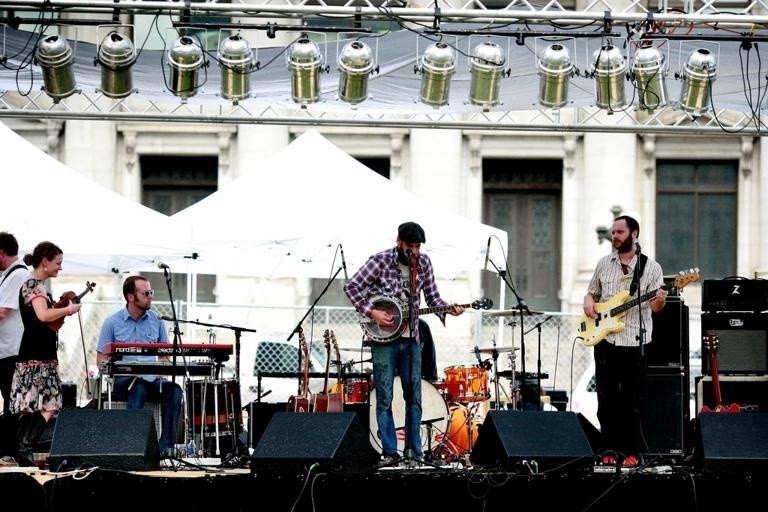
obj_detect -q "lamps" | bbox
[590,45,627,115]
[678,48,718,117]
[35,36,82,104]
[412,41,456,109]
[334,41,374,110]
[95,31,139,99]
[163,36,205,106]
[285,39,328,110]
[215,36,257,106]
[462,41,507,112]
[532,43,574,110]
[631,45,670,116]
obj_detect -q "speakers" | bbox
[48,409,159,472]
[248,412,379,473]
[469,411,593,473]
[692,413,767,473]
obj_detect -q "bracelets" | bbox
[67,307,72,316]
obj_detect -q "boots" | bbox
[10,412,46,468]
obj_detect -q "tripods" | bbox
[158,269,209,472]
[217,332,253,468]
[372,270,444,470]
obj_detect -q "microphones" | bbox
[156,313,177,321]
[339,246,350,284]
[406,249,421,268]
[633,238,641,252]
[483,238,490,269]
[523,315,552,335]
[158,262,169,268]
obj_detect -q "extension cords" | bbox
[593,465,628,474]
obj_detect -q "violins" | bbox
[46,281,97,330]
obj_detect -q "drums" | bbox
[343,377,370,404]
[445,366,488,403]
[366,376,448,458]
[434,403,478,461]
[186,379,244,436]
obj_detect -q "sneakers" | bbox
[159,457,181,468]
[405,457,425,469]
[622,455,639,468]
[601,452,617,467]
[376,455,399,468]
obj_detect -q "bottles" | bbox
[188,439,196,457]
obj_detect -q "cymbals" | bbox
[482,307,545,318]
[472,346,521,353]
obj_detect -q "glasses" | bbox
[143,290,155,298]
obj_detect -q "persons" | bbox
[342,222,465,464]
[0,231,31,465]
[8,241,82,465]
[584,216,668,466]
[95,276,183,455]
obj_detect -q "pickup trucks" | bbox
[83,335,357,418]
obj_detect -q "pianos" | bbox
[109,341,234,356]
[108,361,213,376]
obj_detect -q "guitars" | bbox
[285,328,310,413]
[330,329,345,411]
[698,332,739,414]
[316,332,329,411]
[577,268,703,348]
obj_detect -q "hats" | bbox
[397,221,426,244]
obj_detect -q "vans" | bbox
[565,360,703,430]
